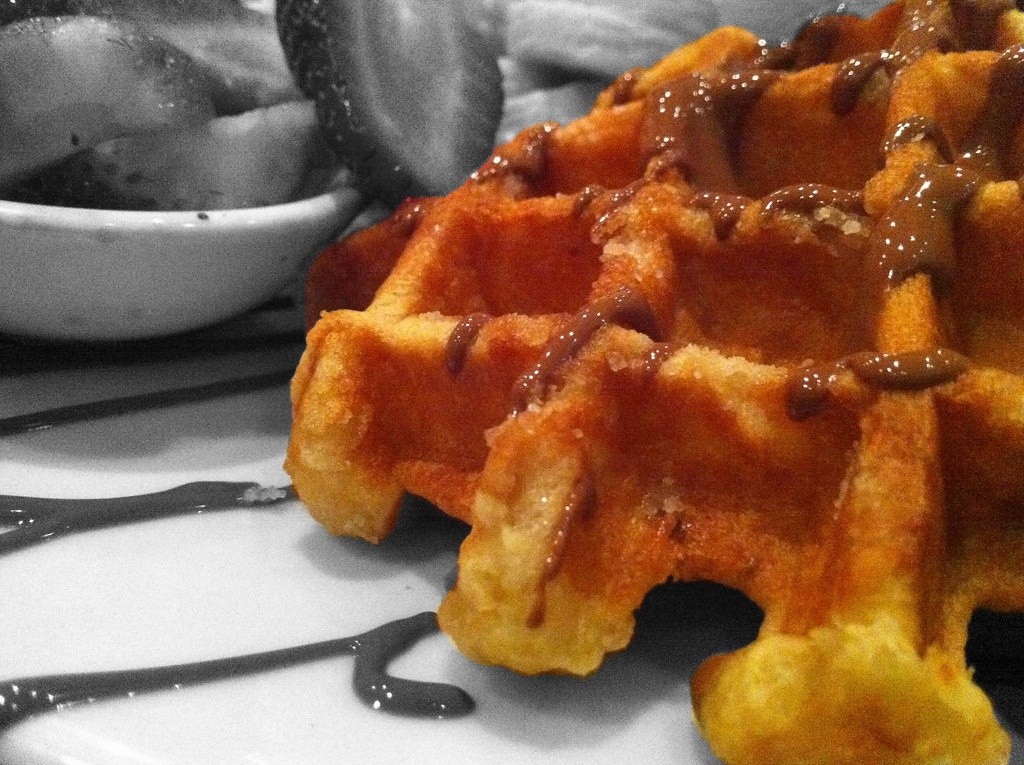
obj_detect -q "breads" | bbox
[280,0,1024,765]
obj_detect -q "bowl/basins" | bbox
[0,18,367,344]
